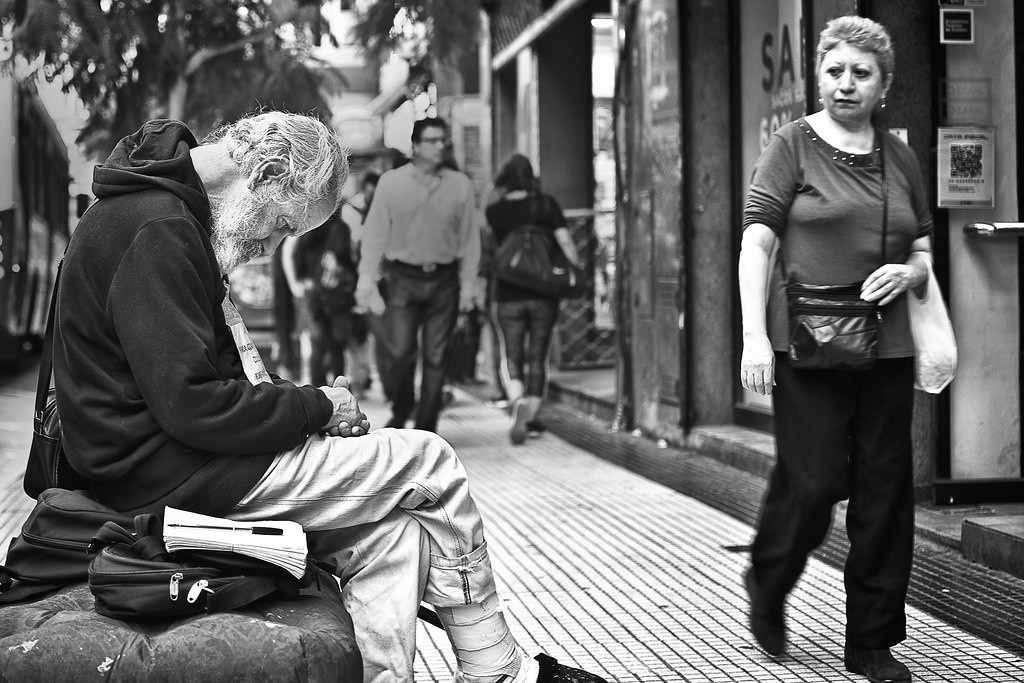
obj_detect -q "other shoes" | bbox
[743,566,787,656]
[535,653,608,683]
[528,421,546,439]
[844,642,911,682]
[509,399,530,444]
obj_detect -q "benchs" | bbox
[0,571,363,683]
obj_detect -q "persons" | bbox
[55,113,610,683]
[293,118,479,433]
[480,153,579,443]
[738,16,932,683]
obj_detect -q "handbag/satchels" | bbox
[495,194,569,297]
[23,399,80,500]
[781,280,884,371]
[0,489,139,607]
[903,249,958,393]
[88,512,321,624]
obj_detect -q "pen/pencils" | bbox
[166,522,284,536]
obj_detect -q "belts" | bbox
[394,260,447,273]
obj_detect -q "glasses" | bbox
[421,136,451,147]
